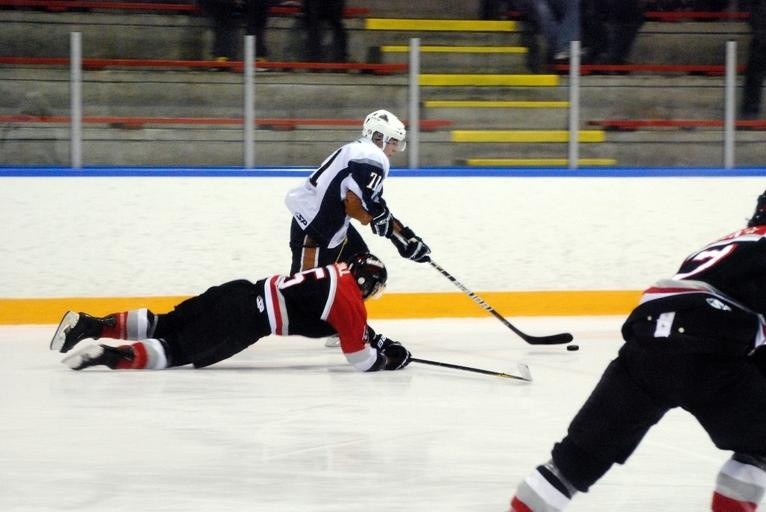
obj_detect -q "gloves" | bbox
[370,334,410,370]
[367,205,393,238]
[390,227,430,262]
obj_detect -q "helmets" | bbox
[361,109,406,151]
[347,254,386,298]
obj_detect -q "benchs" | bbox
[0,3,458,135]
[513,2,764,131]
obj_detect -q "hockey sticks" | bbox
[392,230,574,344]
[410,353,533,386]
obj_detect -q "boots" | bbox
[58,312,115,353]
[72,344,133,370]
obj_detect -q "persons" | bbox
[209,0,274,72]
[578,1,645,74]
[475,0,584,67]
[286,109,431,348]
[50,253,414,372]
[738,2,764,118]
[283,1,349,72]
[499,189,765,512]
